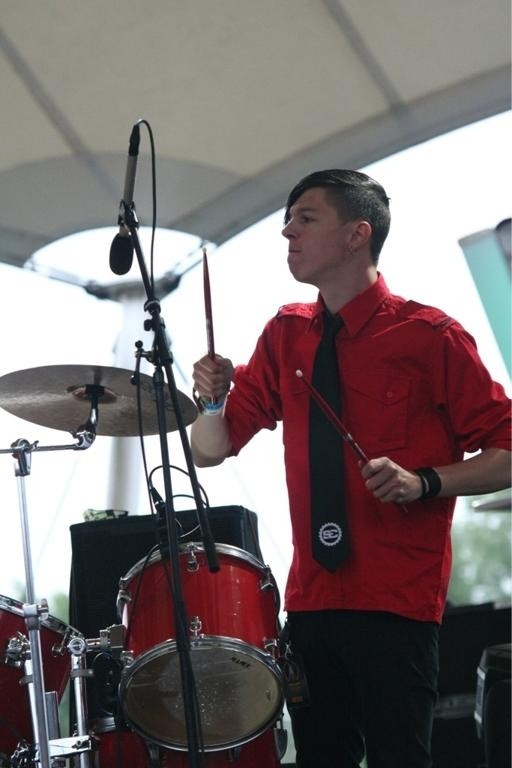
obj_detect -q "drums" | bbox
[88,715,283,767]
[0,593,76,767]
[116,540,288,754]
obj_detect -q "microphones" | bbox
[108,126,140,275]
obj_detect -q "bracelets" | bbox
[192,387,226,416]
[420,465,442,507]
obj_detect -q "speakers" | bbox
[68,505,258,738]
[431,605,511,765]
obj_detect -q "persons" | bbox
[187,169,511,768]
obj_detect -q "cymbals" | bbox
[475,499,511,514]
[0,366,199,437]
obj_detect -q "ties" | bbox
[309,313,351,573]
